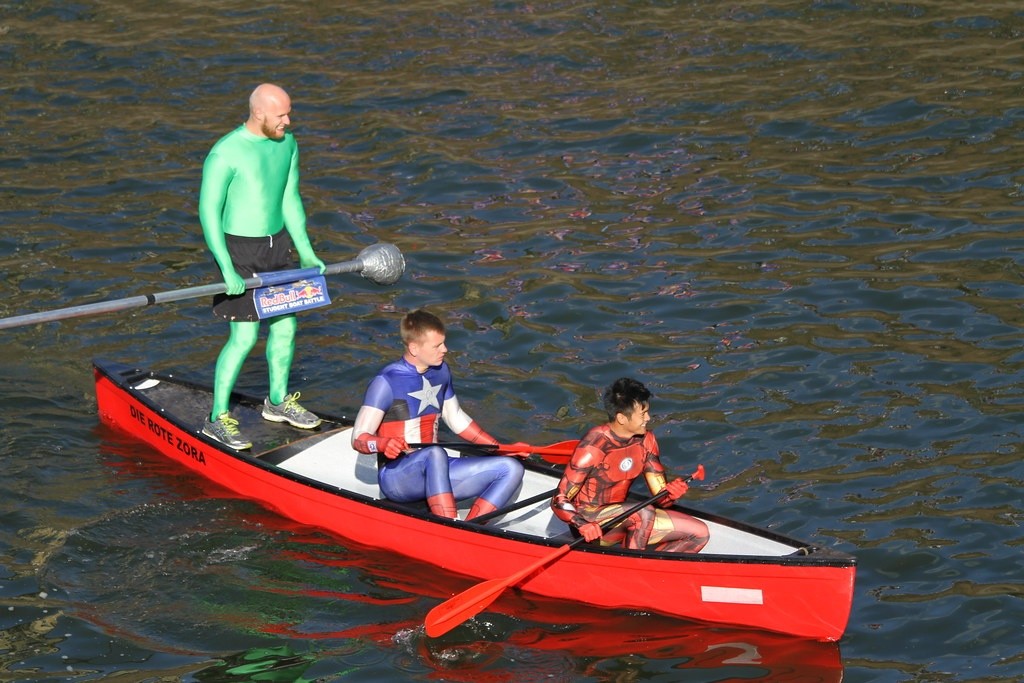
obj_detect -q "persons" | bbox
[551,379,710,554]
[352,310,525,528]
[197,83,326,450]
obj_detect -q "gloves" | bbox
[379,437,411,459]
[664,477,689,501]
[579,520,602,542]
[503,442,532,460]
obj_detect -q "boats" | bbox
[90,357,857,644]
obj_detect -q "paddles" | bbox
[425,463,705,637]
[404,438,584,465]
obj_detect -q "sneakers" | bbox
[202,411,253,451]
[261,391,321,429]
